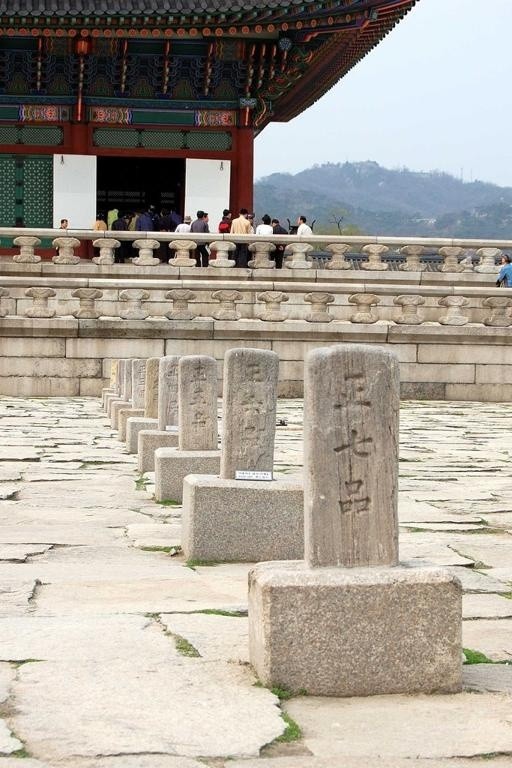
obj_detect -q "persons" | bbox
[497,252,512,288]
[94,203,287,266]
[297,217,312,260]
[55,220,68,255]
